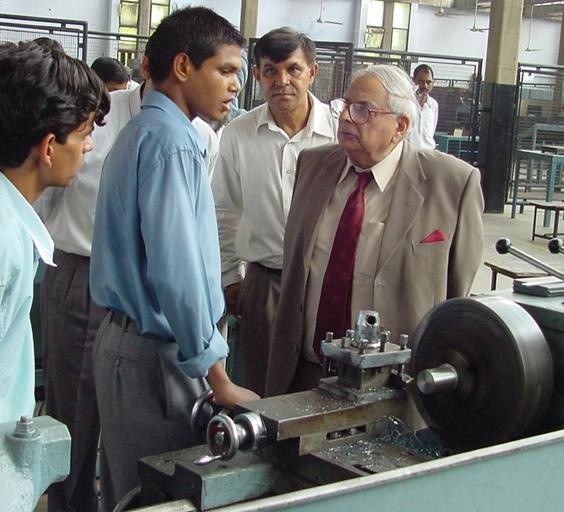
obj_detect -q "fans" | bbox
[309,0,344,26]
[432,1,463,20]
[520,1,545,52]
[469,0,489,33]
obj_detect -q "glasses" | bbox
[328,96,404,125]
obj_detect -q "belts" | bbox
[253,260,283,276]
[111,306,229,345]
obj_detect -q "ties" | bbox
[312,172,373,365]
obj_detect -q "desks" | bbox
[529,201,564,241]
[511,149,564,228]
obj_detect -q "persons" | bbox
[90,6,261,512]
[36,45,221,512]
[410,63,441,149]
[203,49,253,130]
[267,64,488,397]
[120,63,143,91]
[0,37,112,427]
[211,26,342,393]
[89,55,129,93]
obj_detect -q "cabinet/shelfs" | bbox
[438,135,480,167]
